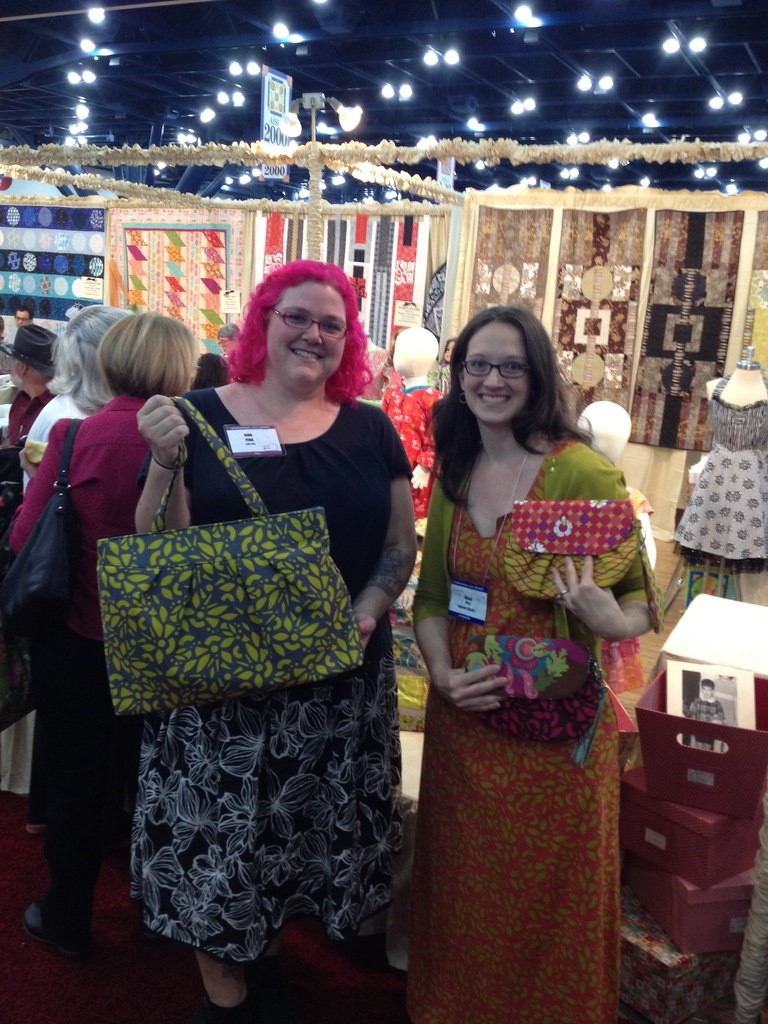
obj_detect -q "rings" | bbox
[560,589,569,594]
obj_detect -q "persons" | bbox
[410,305,665,1024]
[0,308,228,961]
[675,365,767,575]
[217,322,239,361]
[686,680,726,724]
[360,328,387,399]
[380,326,445,521]
[130,259,418,1024]
[575,401,657,573]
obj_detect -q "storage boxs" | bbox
[620,668,768,955]
[618,884,742,1023]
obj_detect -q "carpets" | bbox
[0,196,768,452]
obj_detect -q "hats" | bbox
[0,325,58,378]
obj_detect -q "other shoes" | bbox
[26,816,48,834]
[195,967,264,1024]
[23,902,91,958]
[345,933,386,966]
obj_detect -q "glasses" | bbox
[460,359,531,379]
[269,306,349,339]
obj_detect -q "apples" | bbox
[24,441,48,464]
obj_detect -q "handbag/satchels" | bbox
[0,419,93,731]
[468,661,604,768]
[468,635,586,700]
[99,395,365,718]
[502,499,637,598]
